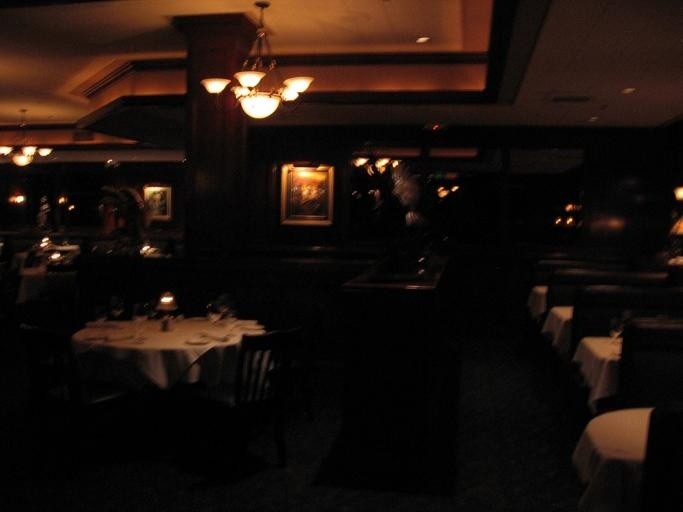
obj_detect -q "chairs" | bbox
[201,326,304,486]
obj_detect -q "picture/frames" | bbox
[280,162,334,225]
[144,185,173,221]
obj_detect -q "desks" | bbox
[72,317,265,399]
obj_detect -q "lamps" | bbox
[0,110,53,167]
[201,0,314,119]
